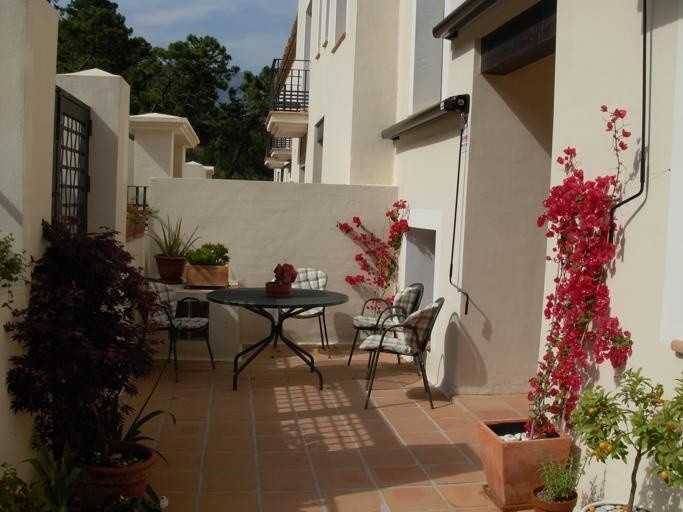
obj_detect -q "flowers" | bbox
[273,263,290,284]
[334,197,410,318]
[523,105,634,436]
[126,205,139,222]
[139,205,153,222]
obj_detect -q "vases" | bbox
[477,415,570,511]
[134,222,145,240]
[126,220,134,241]
[264,281,291,296]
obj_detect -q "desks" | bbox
[209,288,349,394]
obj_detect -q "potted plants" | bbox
[4,216,155,505]
[567,368,682,512]
[527,453,577,509]
[185,242,230,289]
[147,215,200,286]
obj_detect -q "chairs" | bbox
[168,296,218,373]
[273,267,334,360]
[347,282,424,378]
[358,297,444,409]
